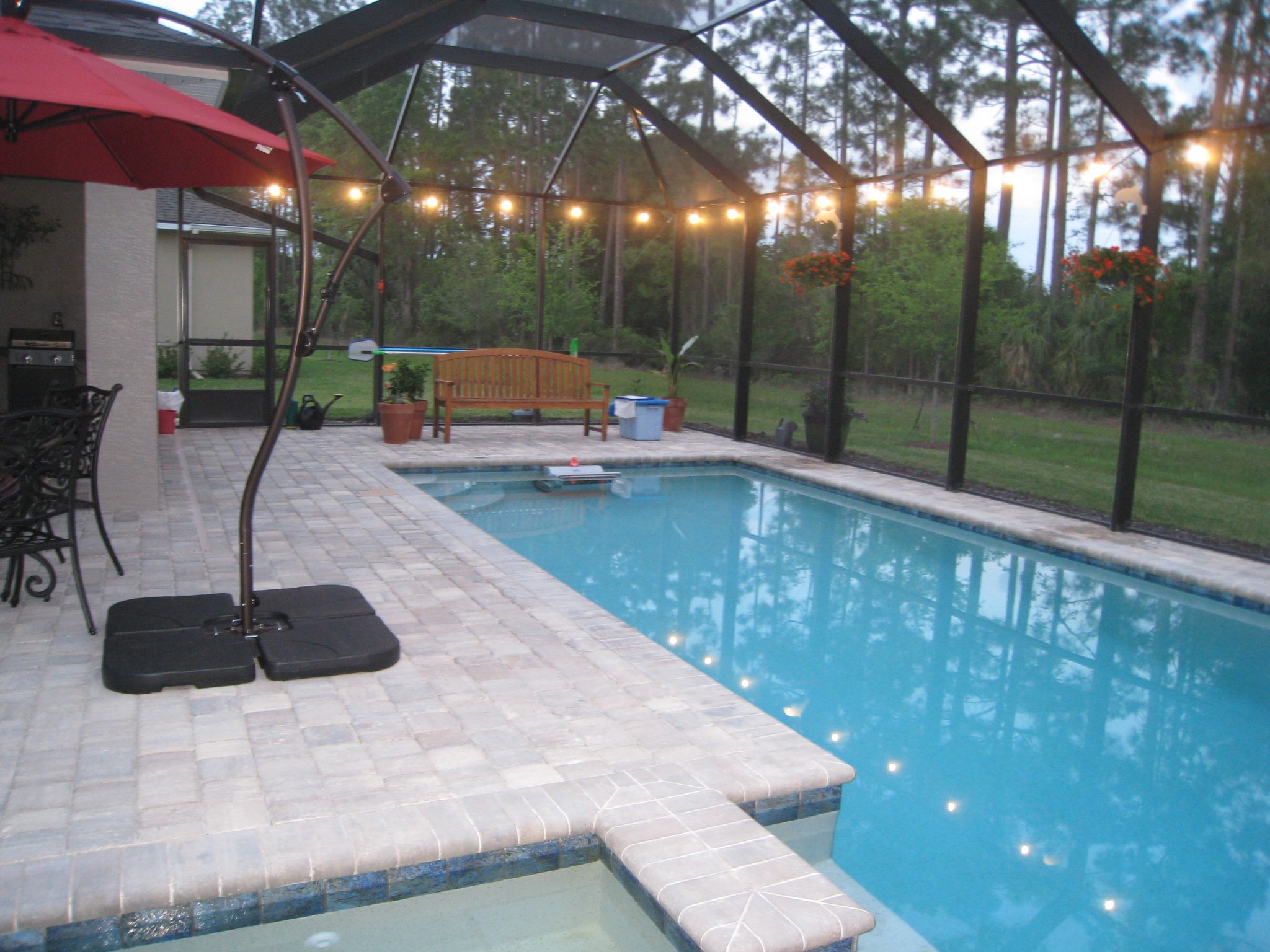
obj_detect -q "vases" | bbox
[1098,271,1129,286]
[802,414,849,455]
[377,401,414,444]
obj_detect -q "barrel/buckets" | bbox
[158,409,177,433]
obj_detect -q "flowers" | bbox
[1062,246,1174,309]
[382,362,407,405]
[779,251,855,297]
[800,375,864,421]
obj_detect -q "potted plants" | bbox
[388,359,432,440]
[651,333,704,431]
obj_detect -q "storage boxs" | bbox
[616,397,671,442]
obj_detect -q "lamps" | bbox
[99,52,230,109]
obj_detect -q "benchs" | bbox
[433,348,611,443]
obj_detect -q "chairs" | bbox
[0,375,124,637]
